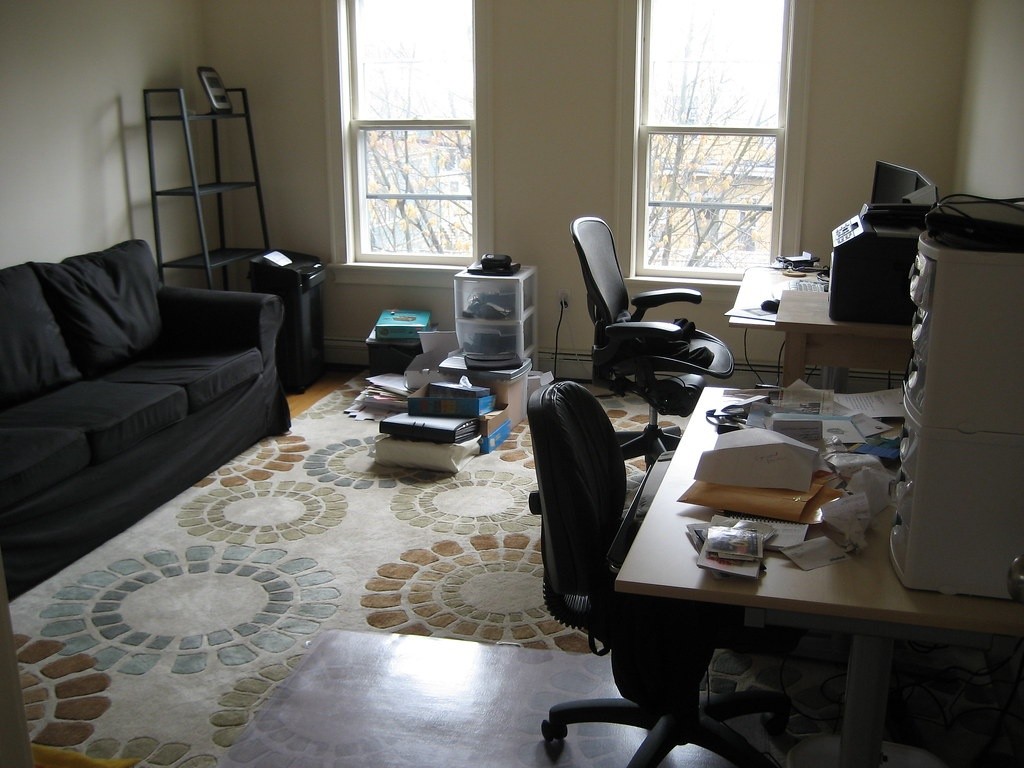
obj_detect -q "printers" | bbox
[826,215,926,326]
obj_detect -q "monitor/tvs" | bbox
[870,160,939,205]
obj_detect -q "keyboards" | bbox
[790,279,825,292]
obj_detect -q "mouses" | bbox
[761,299,780,314]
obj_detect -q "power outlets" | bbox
[559,289,570,312]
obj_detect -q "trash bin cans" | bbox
[247,247,328,395]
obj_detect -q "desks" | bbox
[729,267,912,392]
[776,289,913,391]
[613,386,1024,768]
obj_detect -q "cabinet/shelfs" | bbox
[890,230,1024,600]
[453,266,539,372]
[144,84,270,291]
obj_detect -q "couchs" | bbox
[0,238,291,603]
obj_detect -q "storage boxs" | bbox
[375,309,431,339]
[366,323,440,376]
[439,356,532,431]
[408,382,512,453]
[528,370,554,395]
[405,330,459,388]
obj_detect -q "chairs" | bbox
[527,380,791,768]
[569,217,735,474]
